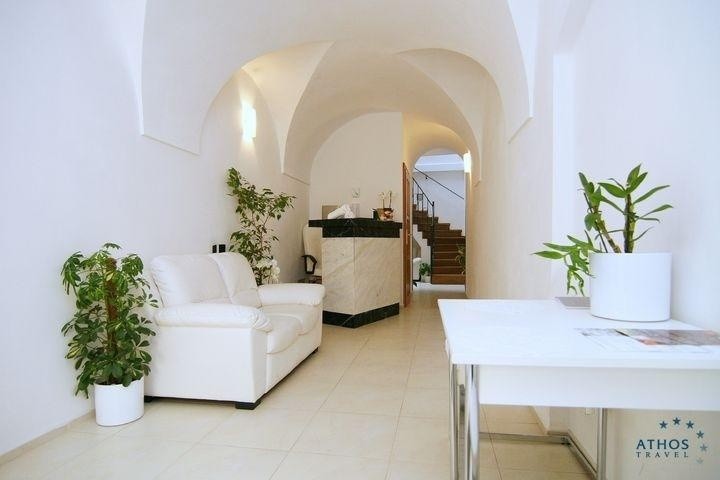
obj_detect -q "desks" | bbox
[436,298,720,479]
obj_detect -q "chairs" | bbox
[302,227,324,284]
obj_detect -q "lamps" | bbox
[239,103,258,139]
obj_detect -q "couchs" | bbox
[100,253,326,411]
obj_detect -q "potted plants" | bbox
[529,162,674,321]
[420,262,432,284]
[62,243,158,427]
